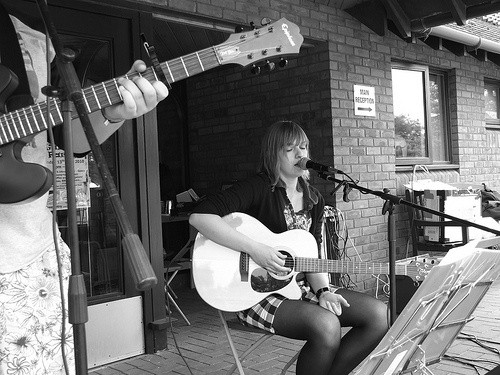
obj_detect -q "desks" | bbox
[162,216,198,289]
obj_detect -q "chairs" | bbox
[164,261,192,326]
[405,189,469,256]
[216,310,301,375]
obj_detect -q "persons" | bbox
[0,12,169,373]
[189,121,391,375]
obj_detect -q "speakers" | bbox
[396,275,423,315]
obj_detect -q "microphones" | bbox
[298,157,344,174]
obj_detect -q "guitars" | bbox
[0,17,305,206]
[192,212,438,313]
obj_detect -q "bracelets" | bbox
[316,287,330,299]
[101,107,123,127]
